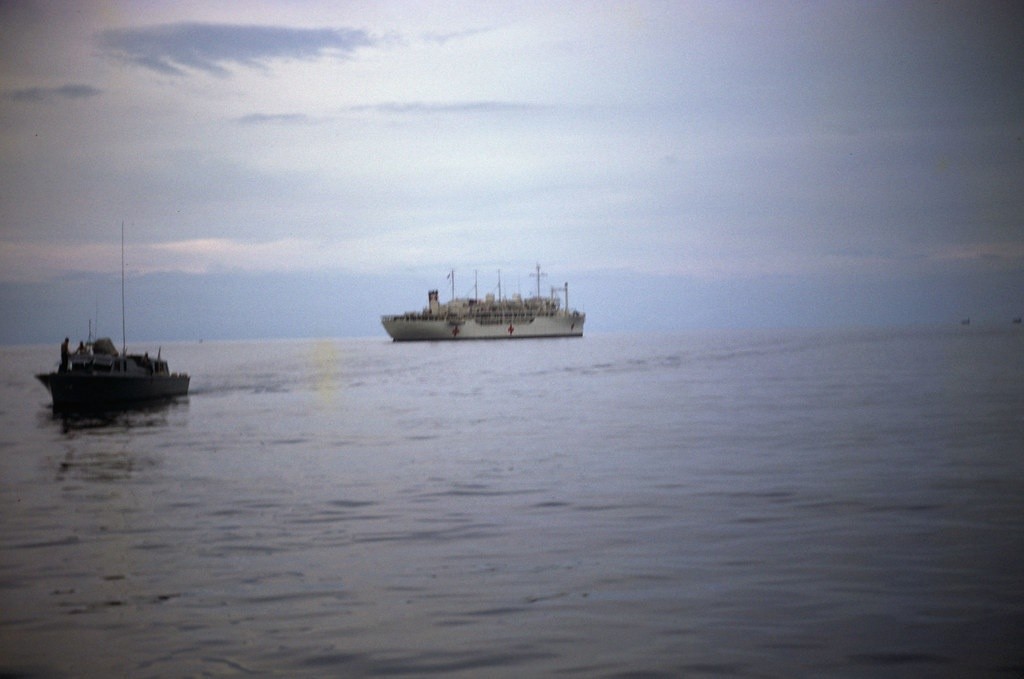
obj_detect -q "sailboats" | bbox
[35,222,191,417]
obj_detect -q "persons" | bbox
[60,338,71,373]
[74,341,85,355]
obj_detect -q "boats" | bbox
[381,264,587,341]
